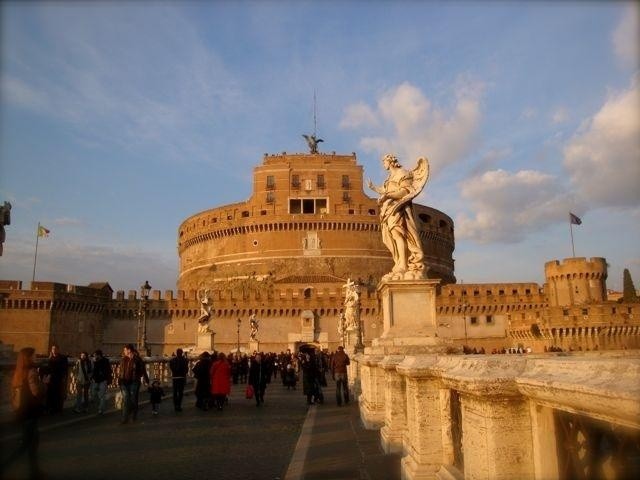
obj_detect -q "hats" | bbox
[152,380,161,387]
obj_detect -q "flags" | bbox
[571,212,582,224]
[38,227,49,239]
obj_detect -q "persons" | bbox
[92,349,114,415]
[338,319,346,347]
[169,348,189,412]
[197,290,214,333]
[250,313,258,341]
[331,346,350,407]
[192,345,336,411]
[145,381,165,415]
[43,345,68,414]
[72,352,91,414]
[0,347,49,479]
[114,344,149,424]
[368,154,415,272]
[344,286,362,330]
[463,344,598,354]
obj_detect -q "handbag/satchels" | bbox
[113,387,127,411]
[245,384,254,400]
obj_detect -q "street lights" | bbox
[139,279,153,351]
[235,318,241,351]
